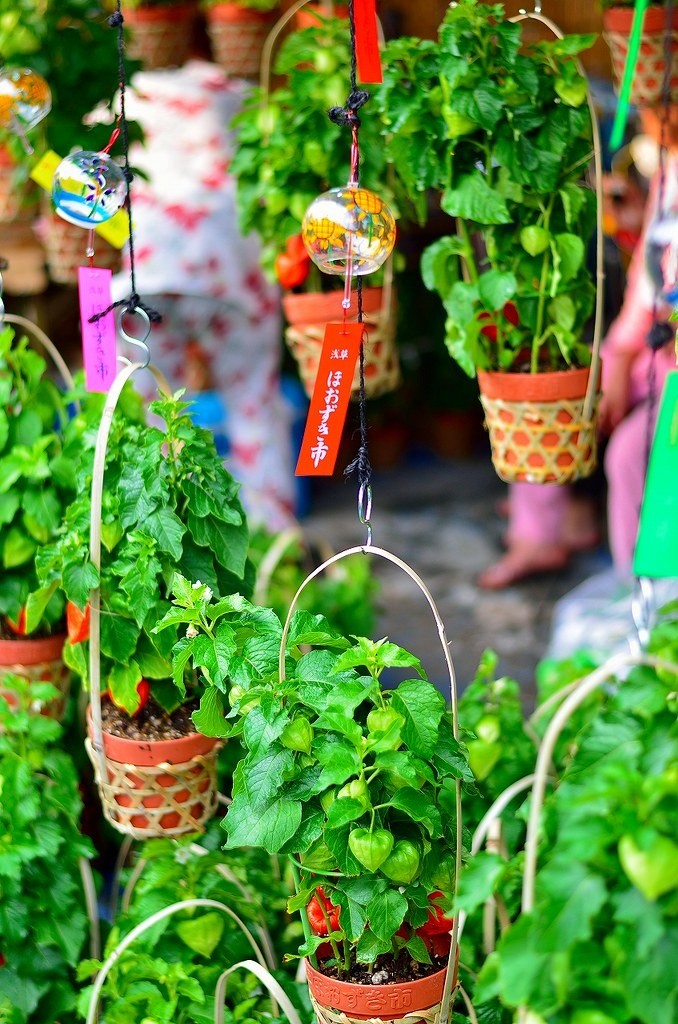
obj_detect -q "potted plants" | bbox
[234,2,427,403]
[0,0,150,282]
[419,0,608,484]
[600,0,677,107]
[0,324,678,1024]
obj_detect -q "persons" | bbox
[86,0,330,580]
[480,106,678,594]
